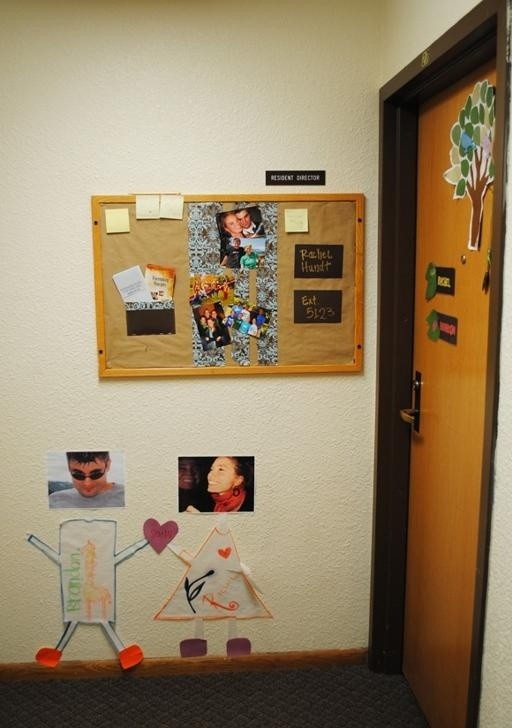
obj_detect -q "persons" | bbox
[190,273,266,351]
[220,211,265,268]
[179,456,253,512]
[49,452,123,507]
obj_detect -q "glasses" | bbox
[68,464,106,481]
